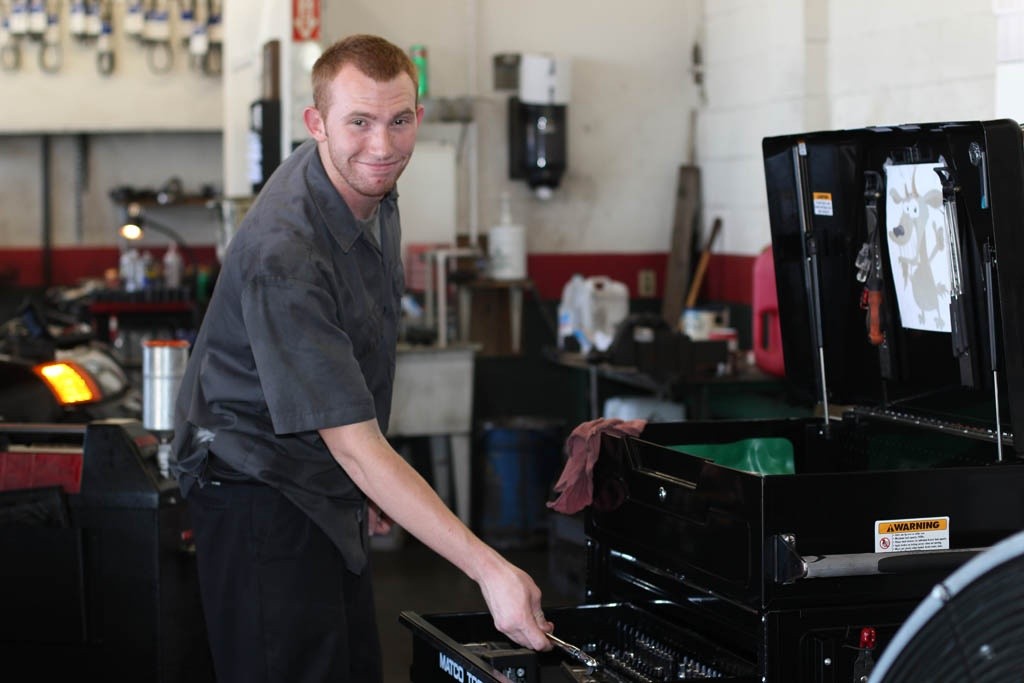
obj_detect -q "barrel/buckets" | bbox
[557,273,629,352]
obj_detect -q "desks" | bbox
[549,350,781,428]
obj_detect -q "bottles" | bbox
[119,241,183,292]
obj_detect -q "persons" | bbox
[169,34,553,681]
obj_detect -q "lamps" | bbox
[118,200,199,301]
